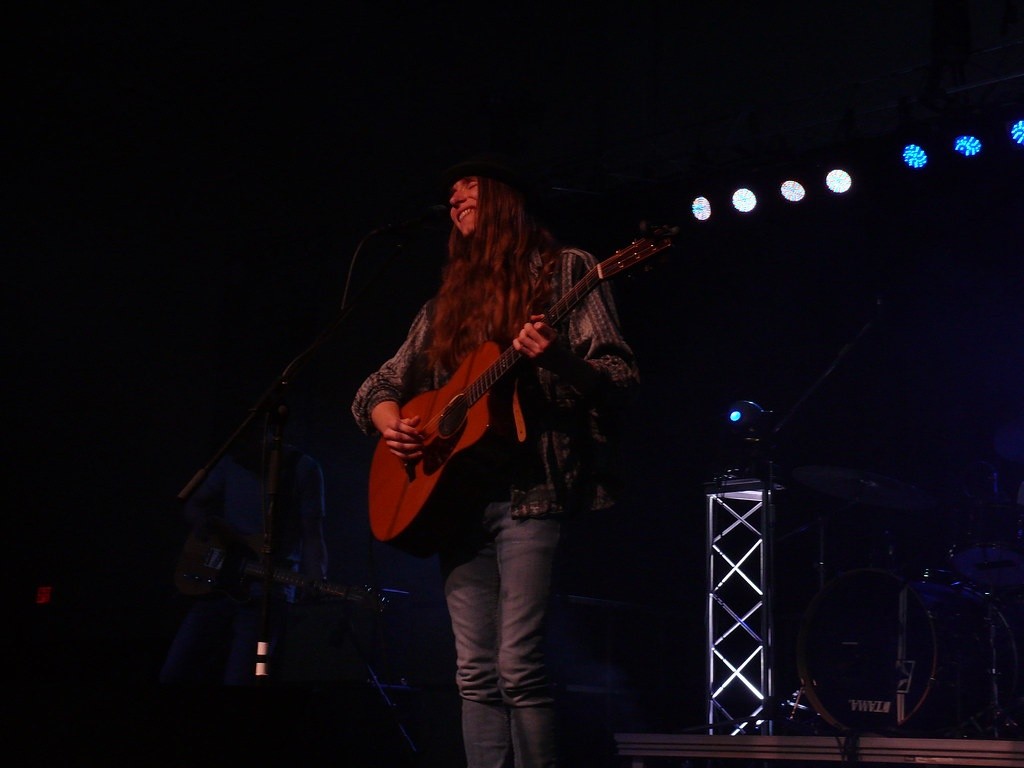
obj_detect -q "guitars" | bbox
[171,512,399,613]
[366,219,682,556]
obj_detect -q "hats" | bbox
[446,153,536,198]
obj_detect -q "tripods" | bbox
[681,315,880,737]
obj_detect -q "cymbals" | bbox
[790,464,943,513]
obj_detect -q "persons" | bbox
[349,150,642,768]
[159,396,328,690]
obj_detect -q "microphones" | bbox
[368,205,449,240]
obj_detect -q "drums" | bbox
[945,497,1024,590]
[791,561,1022,736]
